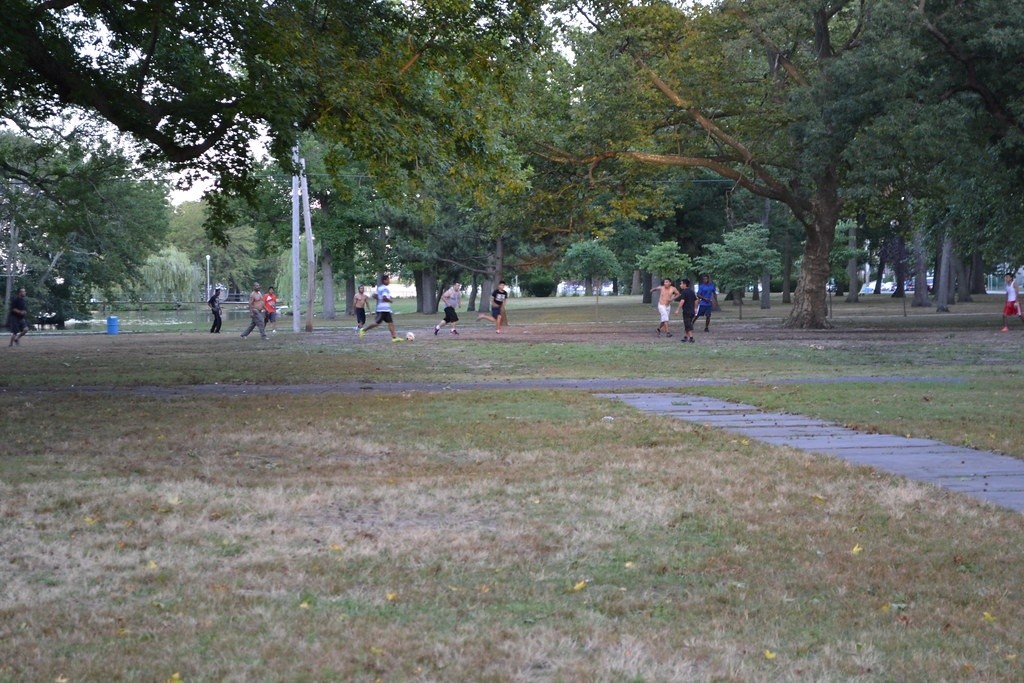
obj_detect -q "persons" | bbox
[649,278,681,338]
[692,274,720,332]
[434,282,464,336]
[359,276,405,343]
[1000,273,1024,331]
[351,285,372,336]
[674,279,699,343]
[475,281,509,334]
[264,288,278,335]
[239,281,270,341]
[7,287,29,346]
[208,288,223,334]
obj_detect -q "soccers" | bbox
[405,331,416,342]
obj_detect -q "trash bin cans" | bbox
[107,316,119,335]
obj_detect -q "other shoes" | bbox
[655,328,660,338]
[359,328,365,342]
[450,330,459,335]
[666,332,673,337]
[689,337,694,342]
[476,313,482,322]
[353,327,358,337]
[692,326,694,331]
[681,337,688,341]
[273,330,276,334]
[265,337,270,341]
[241,335,248,341]
[999,327,1008,332]
[704,327,709,332]
[392,336,405,343]
[434,325,438,336]
[496,329,503,334]
[14,339,19,346]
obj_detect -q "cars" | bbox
[825,277,935,294]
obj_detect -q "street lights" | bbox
[205,255,210,301]
